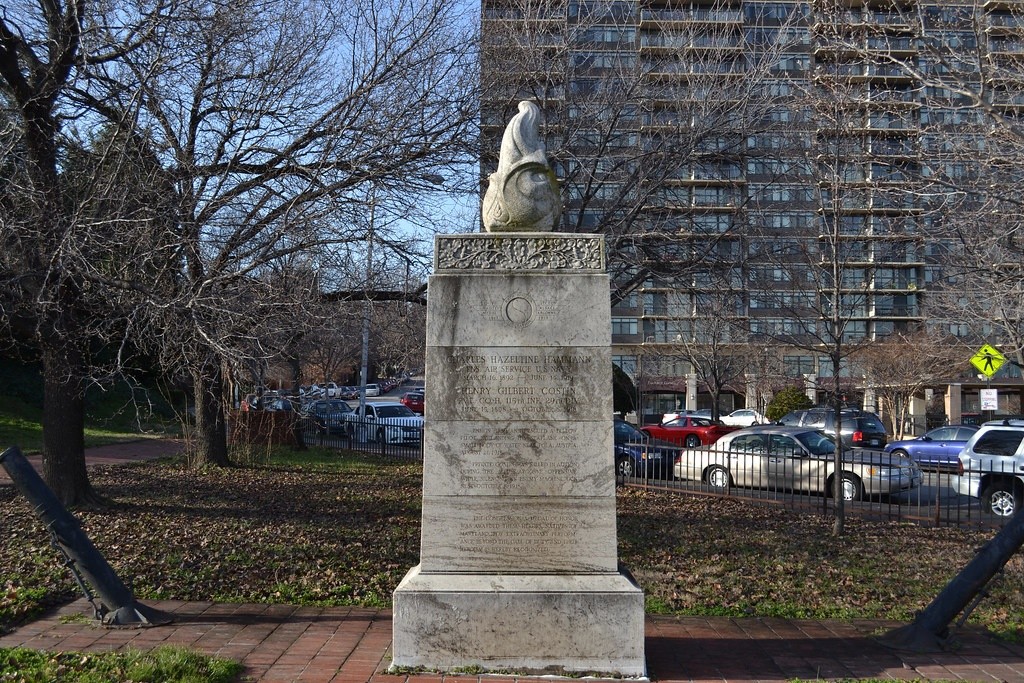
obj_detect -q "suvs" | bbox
[772,408,887,451]
[400,393,424,416]
[952,417,1024,520]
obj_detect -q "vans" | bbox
[366,383,379,397]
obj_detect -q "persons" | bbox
[396,380,401,391]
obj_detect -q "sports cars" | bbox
[639,417,741,448]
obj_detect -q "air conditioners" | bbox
[822,255,829,261]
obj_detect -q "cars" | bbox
[662,410,696,423]
[884,424,981,468]
[613,419,679,477]
[241,387,304,418]
[413,387,425,394]
[348,386,361,400]
[302,385,321,400]
[308,400,353,436]
[674,425,924,501]
[378,379,397,395]
[719,407,774,427]
[685,408,727,420]
[339,386,351,399]
[343,402,424,445]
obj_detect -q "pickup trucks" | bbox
[318,382,341,399]
